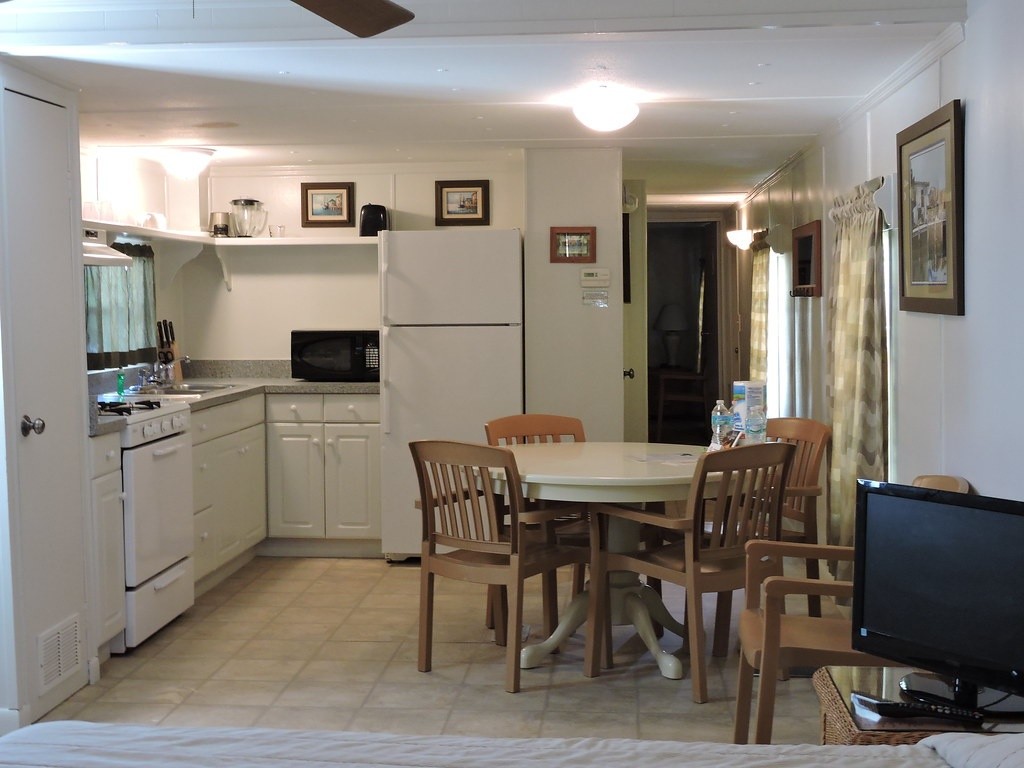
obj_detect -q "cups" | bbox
[268,224,285,239]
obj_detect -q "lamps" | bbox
[654,304,691,373]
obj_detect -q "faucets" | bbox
[137,356,191,385]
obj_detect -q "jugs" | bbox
[228,197,266,238]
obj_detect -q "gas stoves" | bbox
[98,400,191,449]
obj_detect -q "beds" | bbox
[0,720,1024,766]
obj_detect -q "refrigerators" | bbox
[376,226,524,562]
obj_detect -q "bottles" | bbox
[117,366,124,395]
[711,399,767,450]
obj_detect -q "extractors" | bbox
[80,227,133,267]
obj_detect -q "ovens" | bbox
[121,431,195,648]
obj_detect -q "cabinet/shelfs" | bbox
[88,435,128,646]
[189,389,266,599]
[264,393,383,559]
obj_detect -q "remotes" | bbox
[876,702,984,727]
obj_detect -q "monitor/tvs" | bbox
[852,478,1024,718]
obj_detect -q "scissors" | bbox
[158,351,173,366]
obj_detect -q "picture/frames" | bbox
[434,180,490,227]
[788,221,822,297]
[549,224,596,264]
[893,100,965,319]
[300,182,356,228]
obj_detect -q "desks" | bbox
[811,655,1024,744]
[468,443,774,680]
[657,373,710,440]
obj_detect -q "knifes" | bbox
[157,320,176,349]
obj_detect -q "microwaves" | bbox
[291,329,380,382]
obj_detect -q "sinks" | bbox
[163,384,235,390]
[122,389,210,396]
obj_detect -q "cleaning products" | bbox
[116,365,125,395]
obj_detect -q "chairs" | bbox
[702,417,833,659]
[409,437,561,694]
[604,444,795,704]
[485,413,607,630]
[734,474,971,742]
